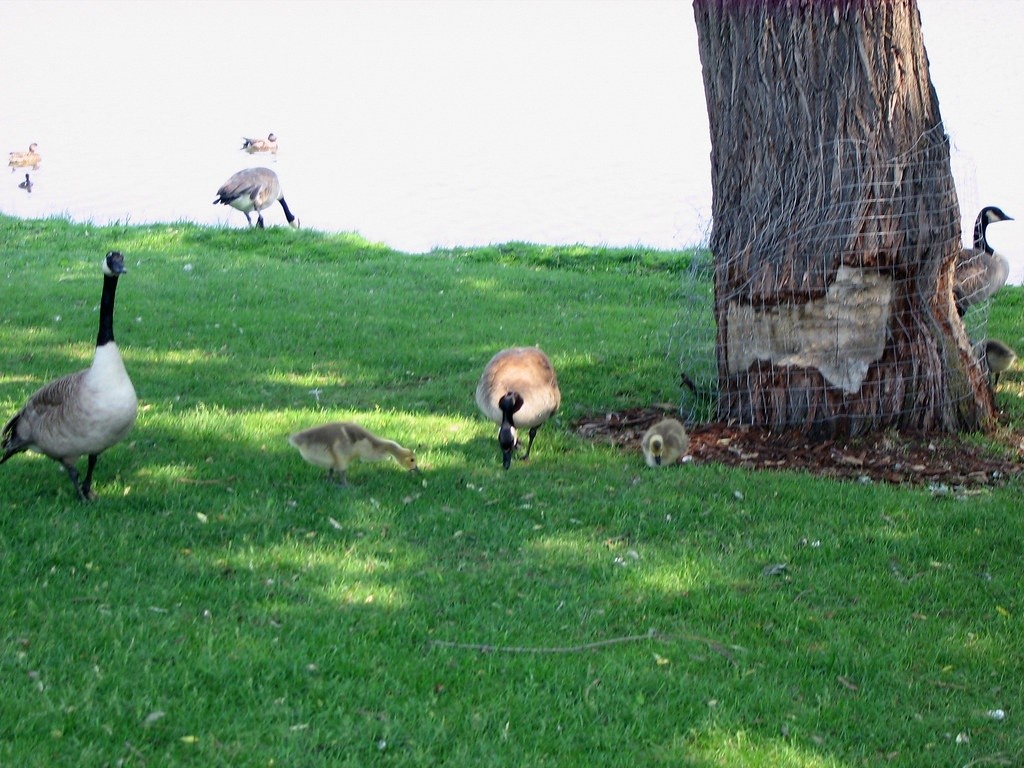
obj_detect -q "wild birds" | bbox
[641,418,691,469]
[953,207,1015,389]
[477,344,562,475]
[289,423,422,486]
[0,251,139,507]
[212,166,301,231]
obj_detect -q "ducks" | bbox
[7,143,42,189]
[240,133,278,152]
